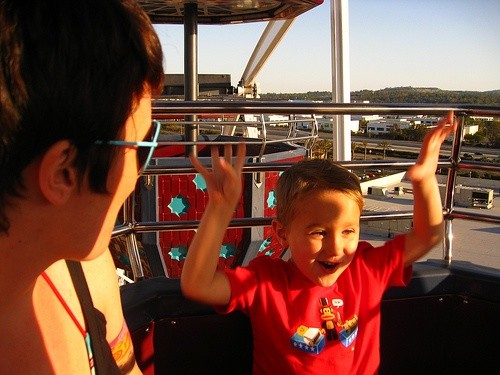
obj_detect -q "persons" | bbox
[181,109,459,375]
[0,0,163,375]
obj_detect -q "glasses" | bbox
[95,119,162,176]
